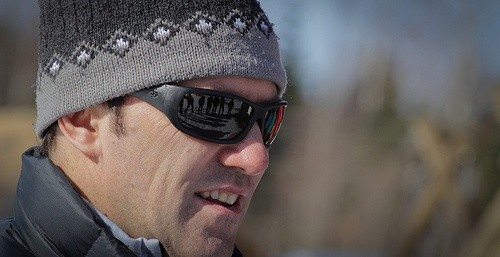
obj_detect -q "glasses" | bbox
[129,84,288,150]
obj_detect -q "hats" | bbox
[35,0,288,139]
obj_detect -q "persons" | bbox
[178,92,235,116]
[1,1,288,257]
